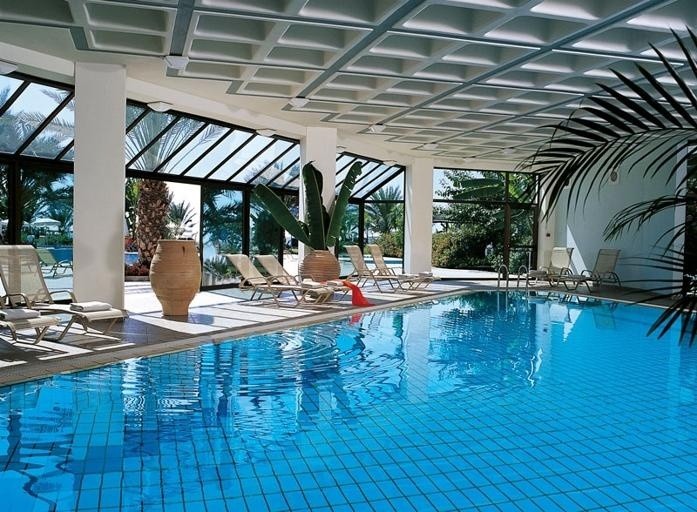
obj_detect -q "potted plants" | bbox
[251,158,364,281]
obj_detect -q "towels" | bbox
[397,273,415,281]
[568,273,586,279]
[530,269,547,277]
[68,300,112,313]
[0,307,40,322]
[325,280,344,287]
[299,281,322,289]
[418,271,434,278]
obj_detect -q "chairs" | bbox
[526,245,576,287]
[558,248,623,294]
[368,242,441,292]
[32,246,72,277]
[344,245,420,293]
[0,240,128,339]
[527,292,620,331]
[217,252,331,309]
[253,254,350,315]
[0,288,61,347]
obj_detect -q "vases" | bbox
[147,238,202,320]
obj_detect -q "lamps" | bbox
[335,122,516,169]
[161,52,191,70]
[254,126,276,139]
[287,95,309,108]
[145,99,173,114]
[0,59,18,76]
